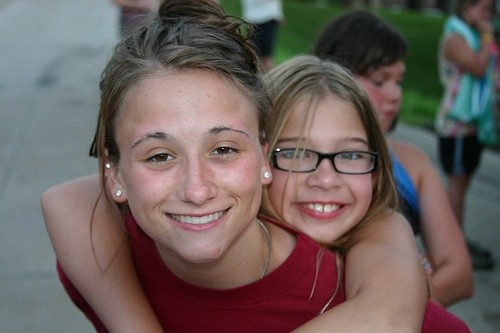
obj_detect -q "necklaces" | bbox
[257,218,272,279]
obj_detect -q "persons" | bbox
[56,0,471,333]
[434,0,500,268]
[310,10,475,309]
[240,0,285,73]
[40,55,428,333]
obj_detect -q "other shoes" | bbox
[466,244,494,271]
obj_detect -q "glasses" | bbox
[270,148,379,175]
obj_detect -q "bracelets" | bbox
[480,33,494,44]
